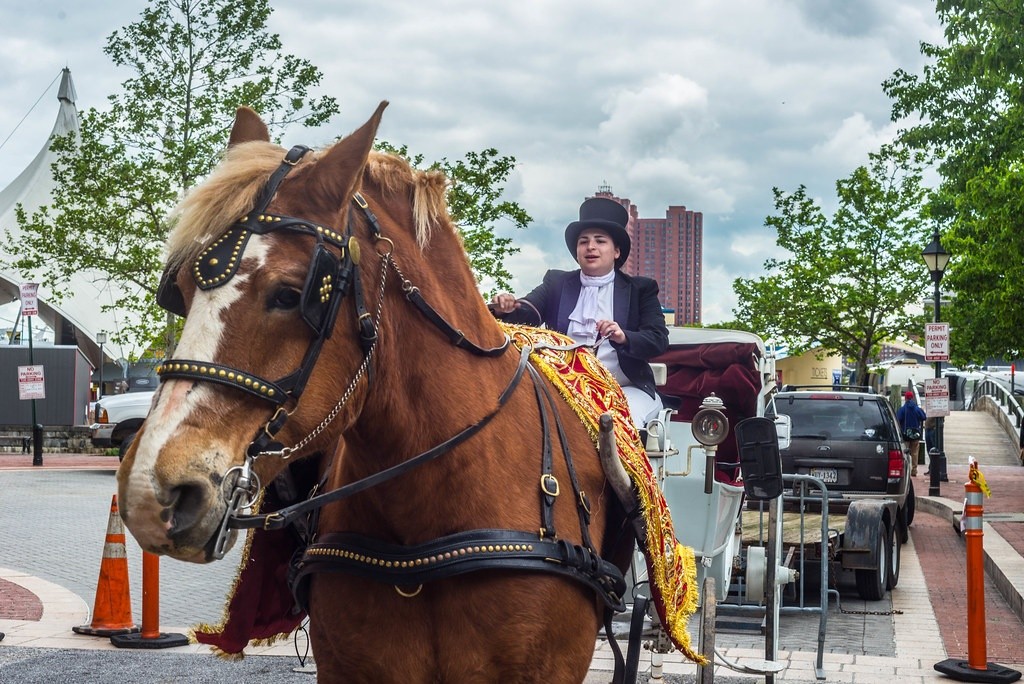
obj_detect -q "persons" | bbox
[894,391,927,479]
[483,194,667,639]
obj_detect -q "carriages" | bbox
[115,99,802,684]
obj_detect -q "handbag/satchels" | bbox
[904,427,922,441]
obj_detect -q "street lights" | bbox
[920,227,954,480]
[97,331,107,400]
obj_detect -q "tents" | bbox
[0,64,218,383]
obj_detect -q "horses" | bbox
[116,101,641,684]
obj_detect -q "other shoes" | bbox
[924,470,930,475]
[911,473,916,477]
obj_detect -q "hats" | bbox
[905,392,914,400]
[565,199,631,270]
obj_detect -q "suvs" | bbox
[765,384,922,544]
[85,391,158,464]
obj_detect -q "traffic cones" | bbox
[72,493,142,636]
